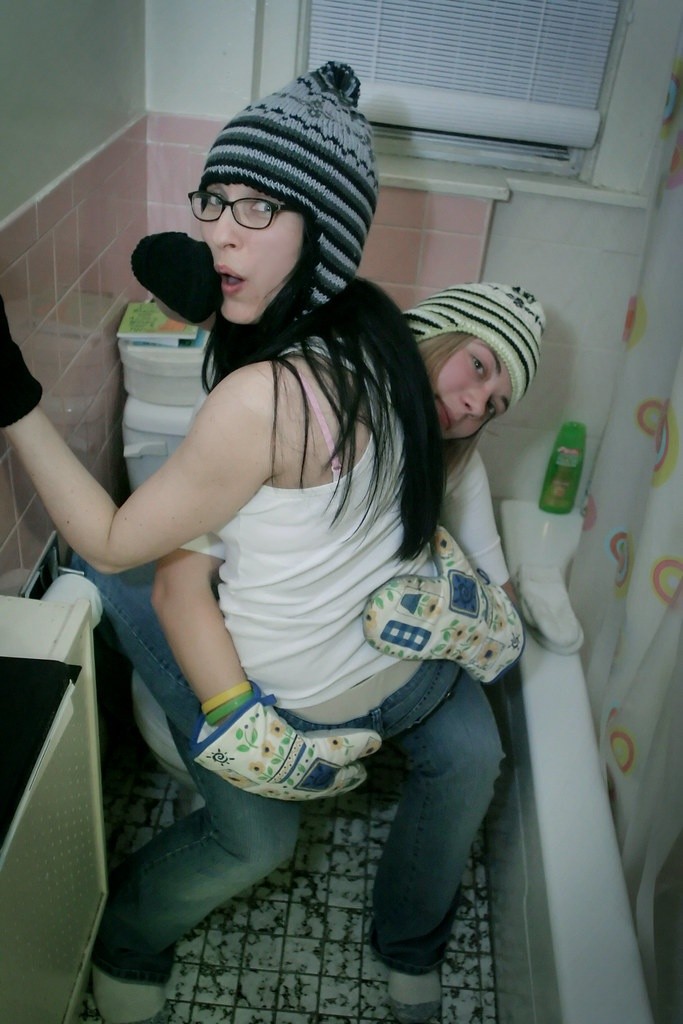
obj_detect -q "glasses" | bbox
[187,191,294,231]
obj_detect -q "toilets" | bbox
[124,371,231,791]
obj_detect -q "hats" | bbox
[402,282,547,416]
[200,61,378,324]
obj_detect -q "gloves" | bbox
[360,526,525,685]
[130,231,222,324]
[188,681,380,801]
[0,296,42,429]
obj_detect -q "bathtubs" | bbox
[490,496,683,1024]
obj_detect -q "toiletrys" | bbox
[536,419,587,513]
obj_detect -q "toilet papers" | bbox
[39,572,105,630]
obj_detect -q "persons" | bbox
[88,282,547,1024]
[0,59,464,752]
